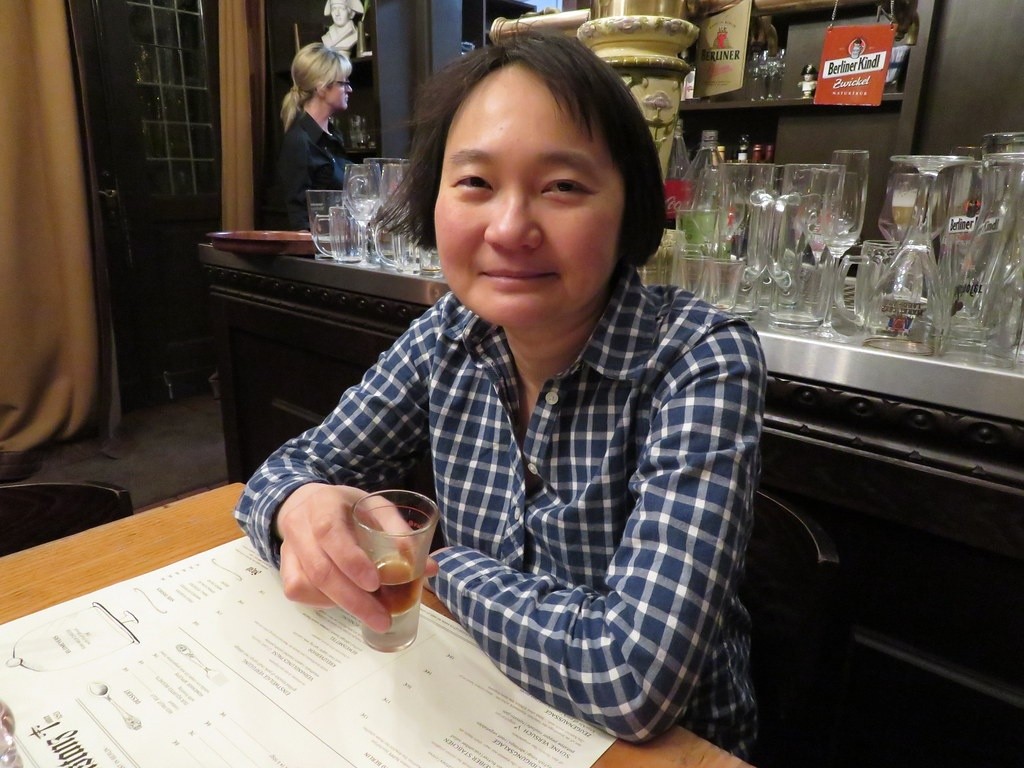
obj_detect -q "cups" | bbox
[328,114,368,149]
[306,189,344,261]
[375,217,442,276]
[637,163,845,329]
[312,205,363,261]
[833,240,905,328]
[364,157,413,205]
[350,489,441,653]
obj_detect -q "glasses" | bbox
[331,79,350,86]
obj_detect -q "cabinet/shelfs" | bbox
[678,0,938,240]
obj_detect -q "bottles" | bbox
[662,118,694,230]
[717,133,776,183]
[926,132,1024,369]
[680,130,728,213]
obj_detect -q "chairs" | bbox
[745,489,840,768]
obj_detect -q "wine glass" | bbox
[864,155,976,357]
[802,150,870,341]
[744,47,788,101]
[381,164,416,275]
[342,163,382,268]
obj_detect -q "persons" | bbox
[272,42,356,229]
[233,30,768,761]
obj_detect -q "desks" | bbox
[0,482,758,768]
